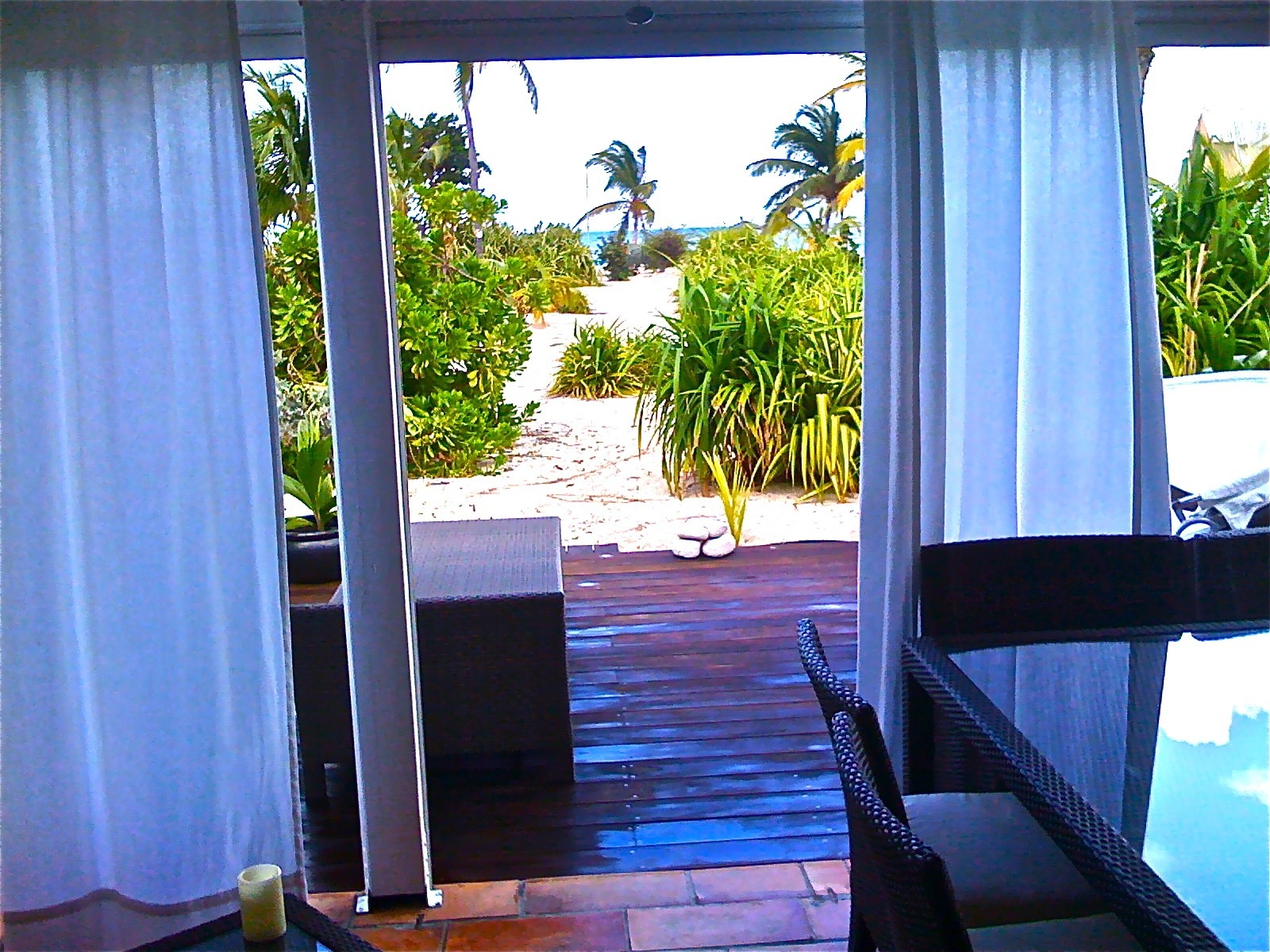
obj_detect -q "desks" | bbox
[289,516,576,811]
[902,619,1270,952]
[123,890,384,952]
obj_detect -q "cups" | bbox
[237,864,287,942]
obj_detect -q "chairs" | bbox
[797,526,1270,952]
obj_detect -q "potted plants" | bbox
[278,414,341,585]
